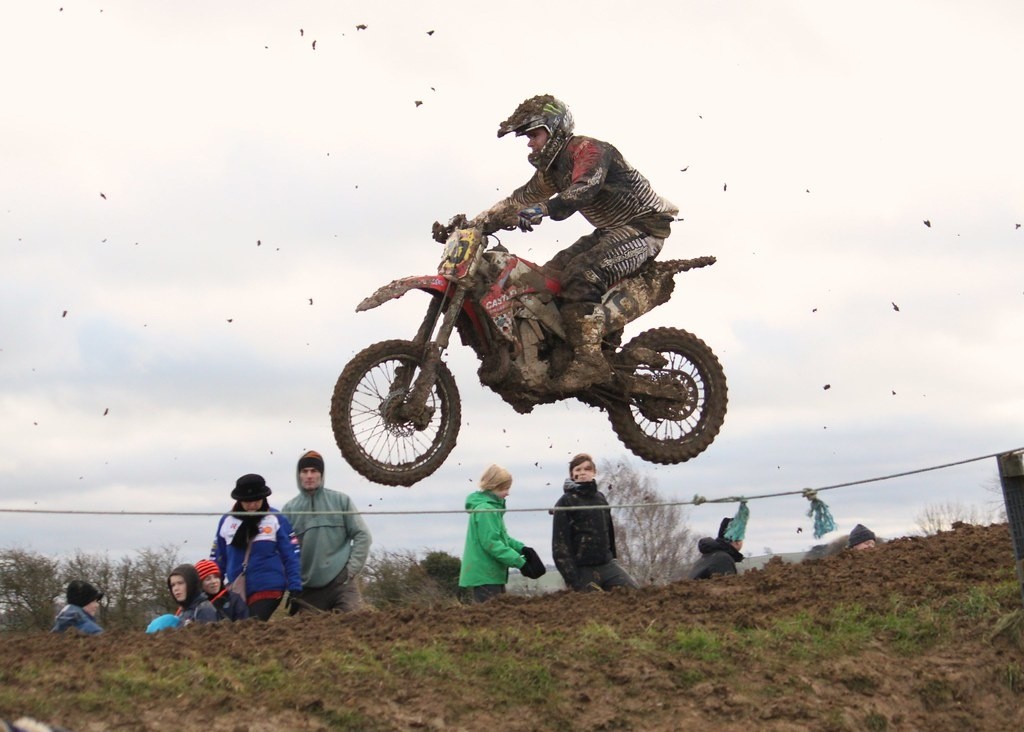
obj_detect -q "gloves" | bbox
[285,592,300,616]
[517,203,544,233]
[520,546,546,579]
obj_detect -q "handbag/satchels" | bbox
[228,571,246,604]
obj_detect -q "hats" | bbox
[717,517,738,542]
[848,524,876,548]
[192,559,222,581]
[298,451,324,475]
[67,581,104,608]
[480,464,512,490]
[231,474,272,502]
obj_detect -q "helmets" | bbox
[497,94,574,174]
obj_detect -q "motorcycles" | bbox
[330,212,728,487]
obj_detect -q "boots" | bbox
[552,313,611,395]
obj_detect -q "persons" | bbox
[434,95,674,399]
[552,454,639,594]
[47,580,105,636]
[848,524,876,550]
[687,518,743,580]
[281,451,372,617]
[168,564,217,626]
[194,559,249,622]
[209,474,303,623]
[460,464,532,604]
